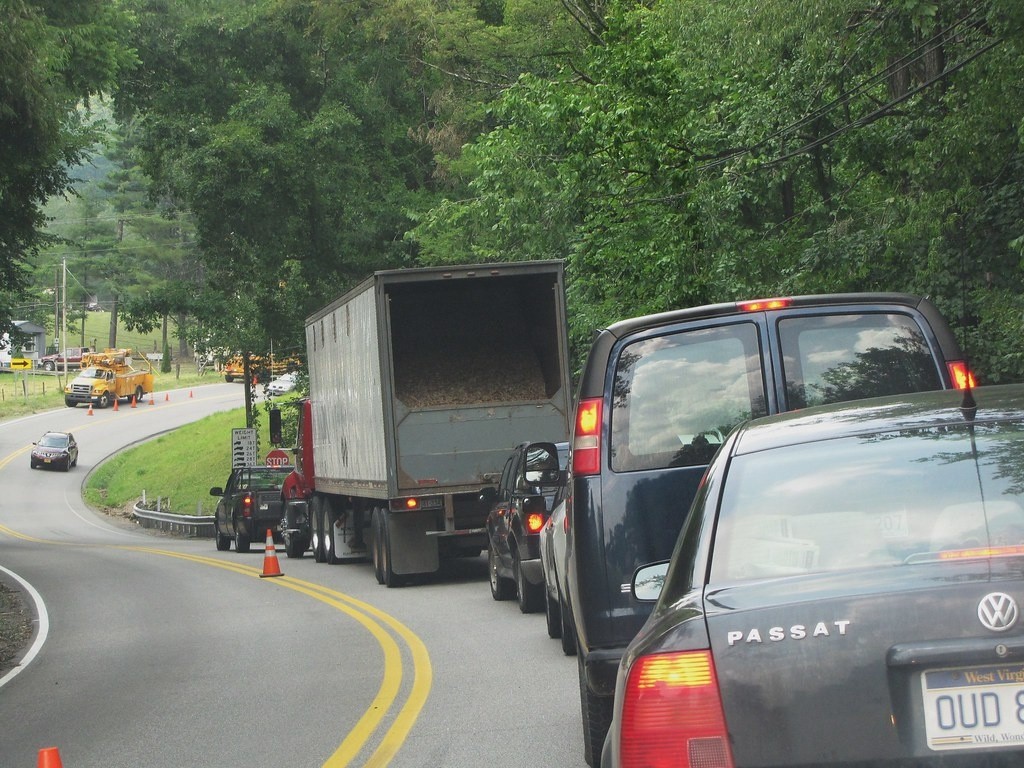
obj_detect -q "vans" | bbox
[526,293,980,768]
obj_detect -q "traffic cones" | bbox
[252,374,258,384]
[148,392,157,406]
[87,401,95,416]
[165,393,170,402]
[112,398,120,412]
[36,743,64,767]
[130,394,139,409]
[189,390,194,398]
[259,528,286,580]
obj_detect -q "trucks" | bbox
[280,256,576,589]
[64,349,153,409]
[225,341,301,383]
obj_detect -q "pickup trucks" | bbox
[209,466,297,552]
[41,347,91,371]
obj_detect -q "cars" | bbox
[486,442,580,659]
[267,369,310,398]
[30,431,79,472]
[599,380,1023,768]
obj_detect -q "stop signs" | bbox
[264,449,289,466]
